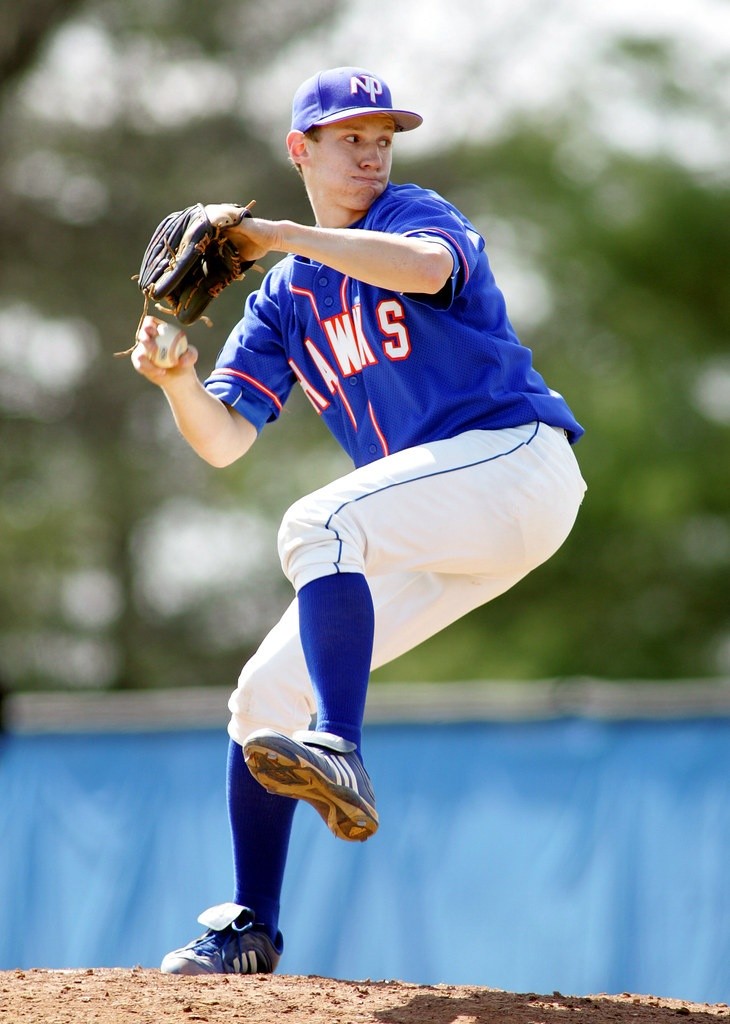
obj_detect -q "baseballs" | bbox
[148,323,191,372]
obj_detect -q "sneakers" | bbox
[243,728,380,841]
[161,902,283,975]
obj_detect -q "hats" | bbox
[291,67,423,133]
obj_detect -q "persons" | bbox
[130,68,586,973]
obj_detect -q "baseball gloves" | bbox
[135,198,256,328]
